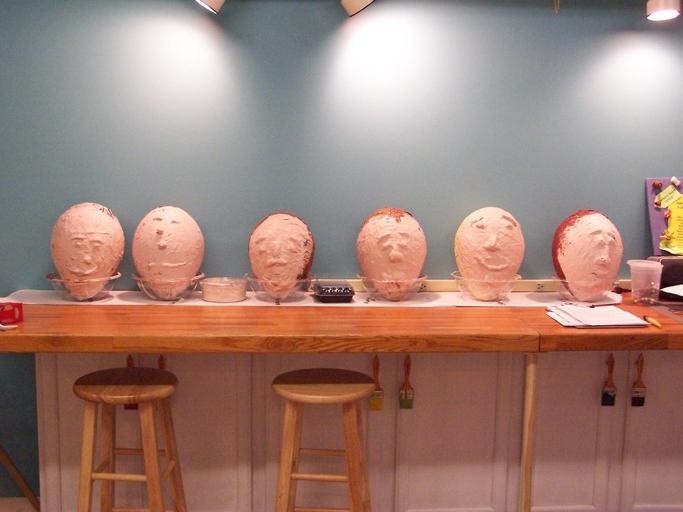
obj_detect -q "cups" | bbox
[627,259,663,306]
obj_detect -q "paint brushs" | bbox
[643,314,663,328]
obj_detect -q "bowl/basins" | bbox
[131,270,204,301]
[46,271,120,301]
[242,271,315,303]
[554,277,620,305]
[357,272,426,301]
[450,271,521,303]
[199,278,247,303]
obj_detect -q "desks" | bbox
[1,287,683,511]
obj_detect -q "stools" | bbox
[1,446,42,512]
[270,367,377,511]
[72,367,187,511]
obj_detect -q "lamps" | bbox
[645,0,680,22]
[195,0,225,16]
[339,0,375,18]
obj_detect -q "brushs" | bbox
[631,352,647,406]
[399,354,415,409]
[601,353,617,406]
[367,354,385,411]
[155,354,167,411]
[124,354,139,410]
